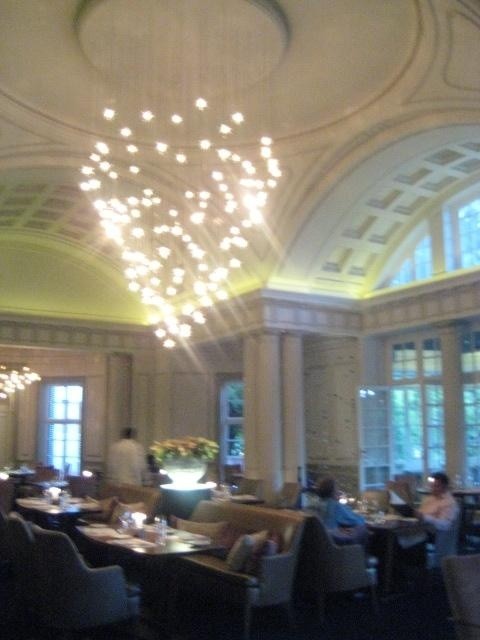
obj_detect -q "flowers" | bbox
[149,434,220,461]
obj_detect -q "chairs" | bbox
[0,455,480,640]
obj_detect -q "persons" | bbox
[303,477,380,599]
[105,424,156,489]
[409,471,461,546]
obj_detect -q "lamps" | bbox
[70,0,288,351]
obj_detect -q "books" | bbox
[387,489,414,517]
